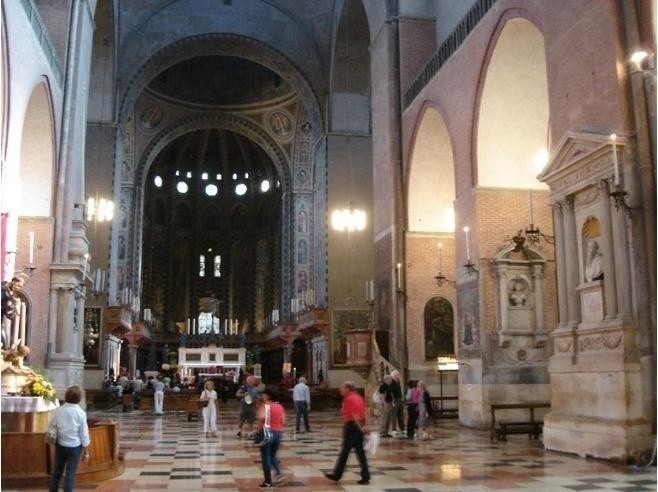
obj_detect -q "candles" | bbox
[465,231,470,259]
[28,232,34,267]
[397,266,401,288]
[610,132,620,187]
[528,187,533,224]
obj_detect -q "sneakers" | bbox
[258,482,273,489]
[235,432,241,439]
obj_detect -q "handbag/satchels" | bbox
[44,424,57,446]
[198,400,208,408]
[254,426,273,447]
[373,385,387,405]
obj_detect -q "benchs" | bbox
[489,402,551,442]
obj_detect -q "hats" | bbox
[258,386,282,400]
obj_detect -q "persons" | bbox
[99,367,206,415]
[200,380,221,439]
[48,384,92,491]
[511,281,527,305]
[325,379,372,484]
[375,369,437,441]
[291,375,312,433]
[0,276,43,378]
[584,240,604,279]
[235,375,260,439]
[255,384,287,489]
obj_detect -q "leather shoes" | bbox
[357,479,369,485]
[324,472,339,481]
